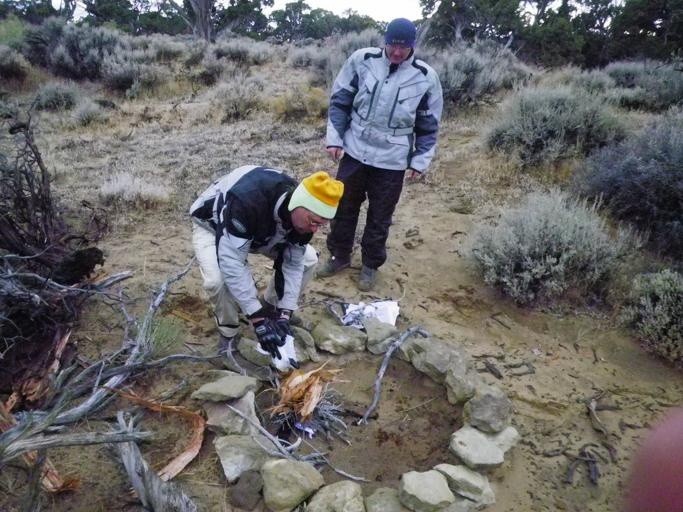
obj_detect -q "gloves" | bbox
[273,318,295,347]
[253,320,282,360]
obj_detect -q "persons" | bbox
[310,15,445,292]
[189,164,349,360]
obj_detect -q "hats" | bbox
[384,17,417,49]
[286,171,347,220]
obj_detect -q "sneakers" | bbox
[259,298,303,325]
[356,263,376,292]
[315,255,351,278]
[215,333,240,356]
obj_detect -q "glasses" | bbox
[303,209,328,226]
